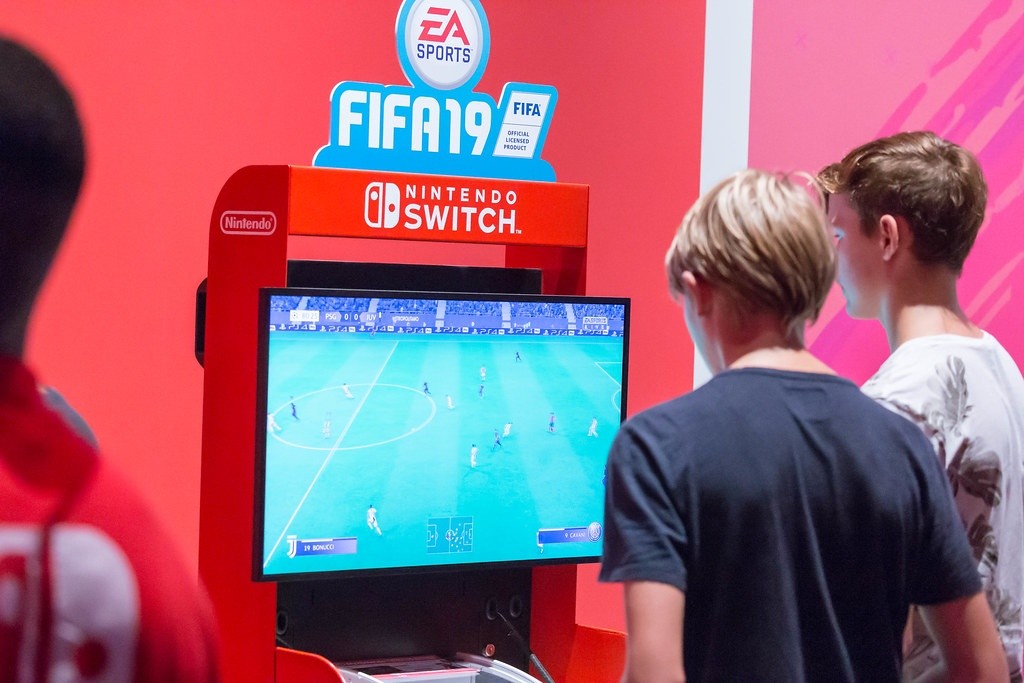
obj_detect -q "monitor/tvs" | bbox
[252,287,631,581]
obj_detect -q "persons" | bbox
[808,130,1024,683]
[265,293,624,552]
[597,169,1010,682]
[0,35,221,683]
